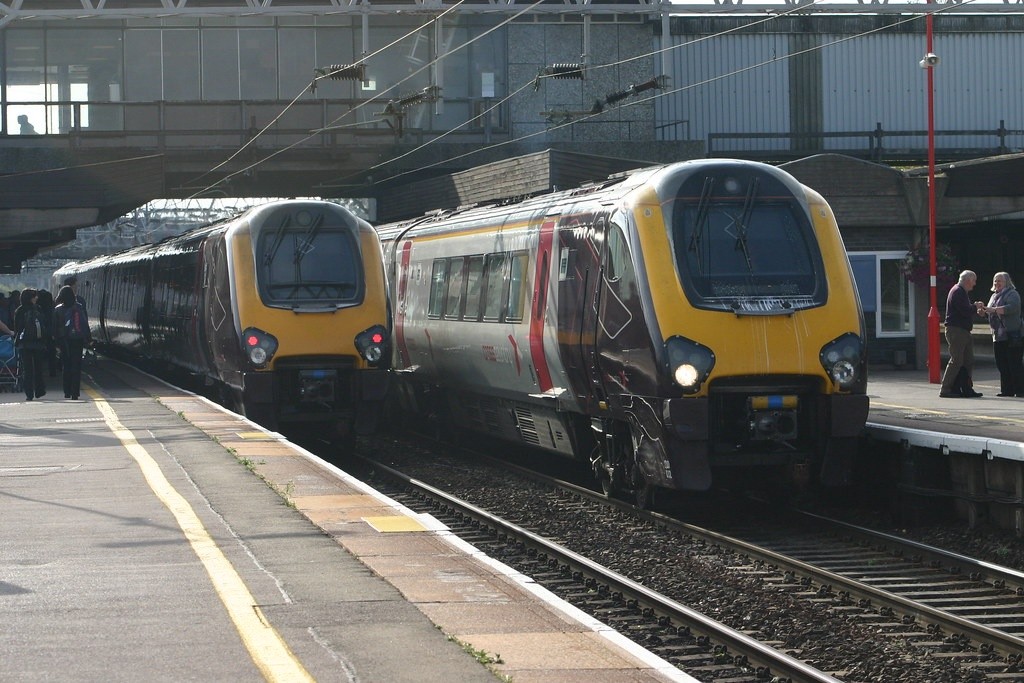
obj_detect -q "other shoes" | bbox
[940,390,963,397]
[36,391,46,398]
[1016,393,1024,397]
[65,392,70,398]
[26,394,34,401]
[960,389,983,397]
[72,392,78,400]
[998,391,1014,396]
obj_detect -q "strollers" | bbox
[0,334,22,393]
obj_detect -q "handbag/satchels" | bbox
[1007,331,1024,348]
[85,343,97,367]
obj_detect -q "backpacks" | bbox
[20,306,48,350]
[59,304,89,337]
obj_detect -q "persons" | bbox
[17,115,38,134]
[0,276,96,400]
[940,269,1024,398]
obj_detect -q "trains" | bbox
[51,199,393,450]
[373,159,870,510]
